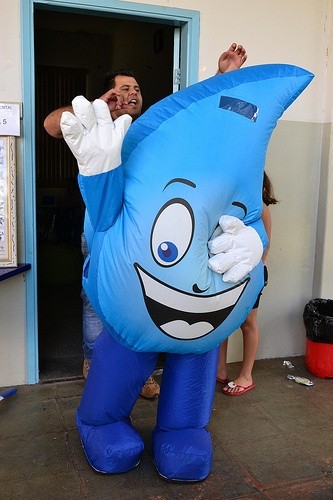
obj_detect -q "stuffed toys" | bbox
[58,64,316,484]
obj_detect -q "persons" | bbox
[215,170,281,397]
[42,43,249,400]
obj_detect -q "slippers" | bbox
[217,378,255,396]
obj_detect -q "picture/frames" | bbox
[0,137,16,268]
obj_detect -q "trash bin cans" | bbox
[304,298,333,379]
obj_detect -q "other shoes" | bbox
[83,359,90,380]
[140,374,161,399]
